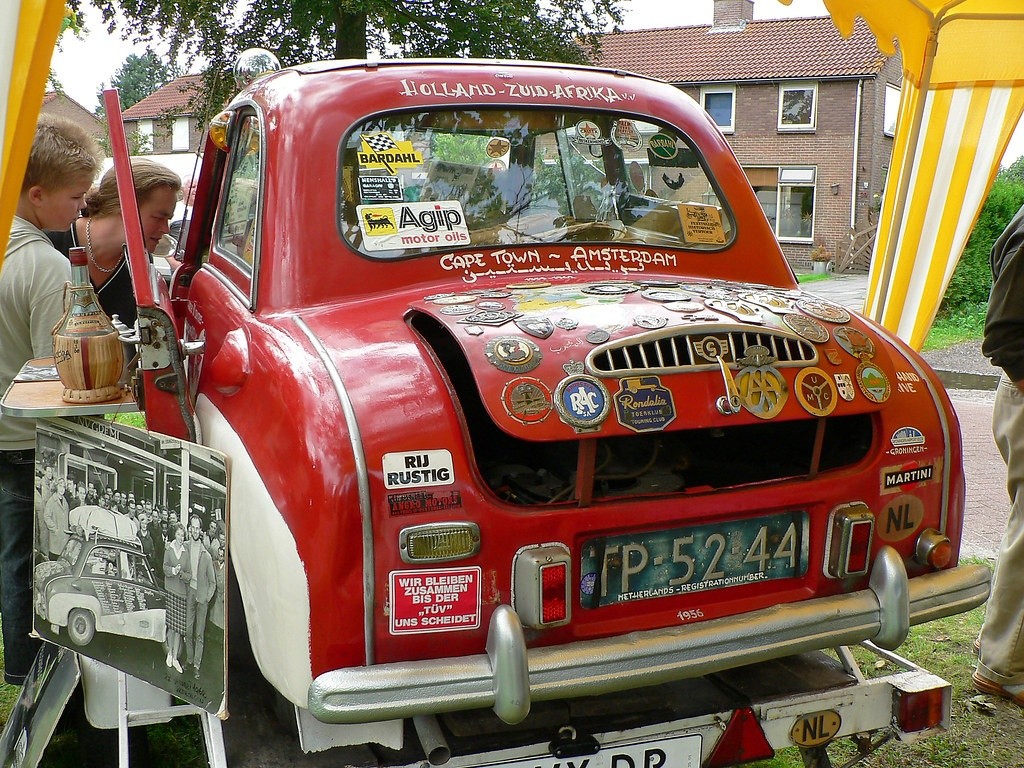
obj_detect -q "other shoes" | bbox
[5,670,27,686]
[973,639,981,656]
[971,670,1024,707]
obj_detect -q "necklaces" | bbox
[86,217,123,272]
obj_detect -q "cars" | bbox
[34,532,165,646]
[100,58,995,767]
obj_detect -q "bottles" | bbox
[57,247,113,338]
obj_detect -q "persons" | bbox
[971,204,1024,710]
[0,113,106,686]
[41,159,181,373]
[34,466,225,679]
